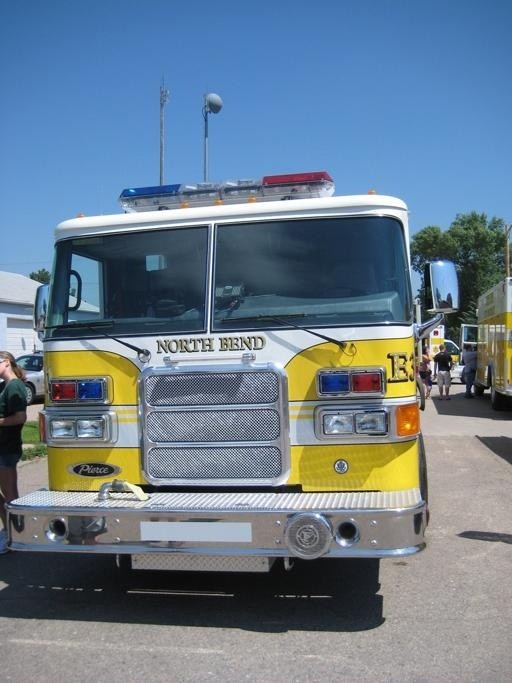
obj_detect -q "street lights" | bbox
[201,93,221,184]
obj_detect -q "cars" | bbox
[1,348,45,404]
[449,362,466,382]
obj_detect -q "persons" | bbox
[0,351,27,554]
[463,344,477,398]
[419,346,432,400]
[433,344,453,400]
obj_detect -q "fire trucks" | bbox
[6,167,464,578]
[456,278,511,412]
[413,323,461,385]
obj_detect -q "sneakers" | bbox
[0,528,9,554]
